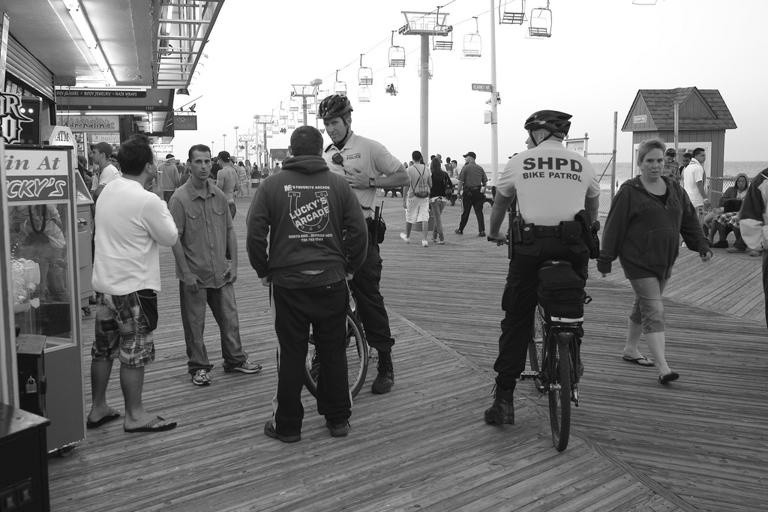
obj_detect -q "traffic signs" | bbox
[470,83,492,92]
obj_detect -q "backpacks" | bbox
[411,165,428,197]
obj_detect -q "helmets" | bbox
[525,110,572,139]
[318,95,353,119]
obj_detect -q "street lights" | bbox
[309,77,324,131]
[221,133,226,150]
[252,113,261,166]
[233,125,239,157]
[211,141,214,155]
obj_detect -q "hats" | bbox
[463,152,476,159]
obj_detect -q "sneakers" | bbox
[192,369,211,385]
[224,358,262,373]
[478,232,487,237]
[713,240,761,256]
[455,229,463,235]
[421,239,445,247]
[400,232,410,242]
[264,421,350,442]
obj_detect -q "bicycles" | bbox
[483,231,586,448]
[296,282,376,407]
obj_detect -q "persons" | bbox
[739,168,766,297]
[704,173,763,256]
[482,110,601,426]
[384,150,462,248]
[162,152,281,220]
[90,142,121,304]
[89,136,177,431]
[245,127,368,443]
[661,148,711,229]
[455,152,488,237]
[168,145,264,386]
[595,141,715,385]
[308,93,411,395]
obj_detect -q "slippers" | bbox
[623,354,655,366]
[125,416,176,432]
[87,409,120,428]
[658,372,678,384]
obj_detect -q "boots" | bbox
[372,350,394,394]
[485,384,514,425]
